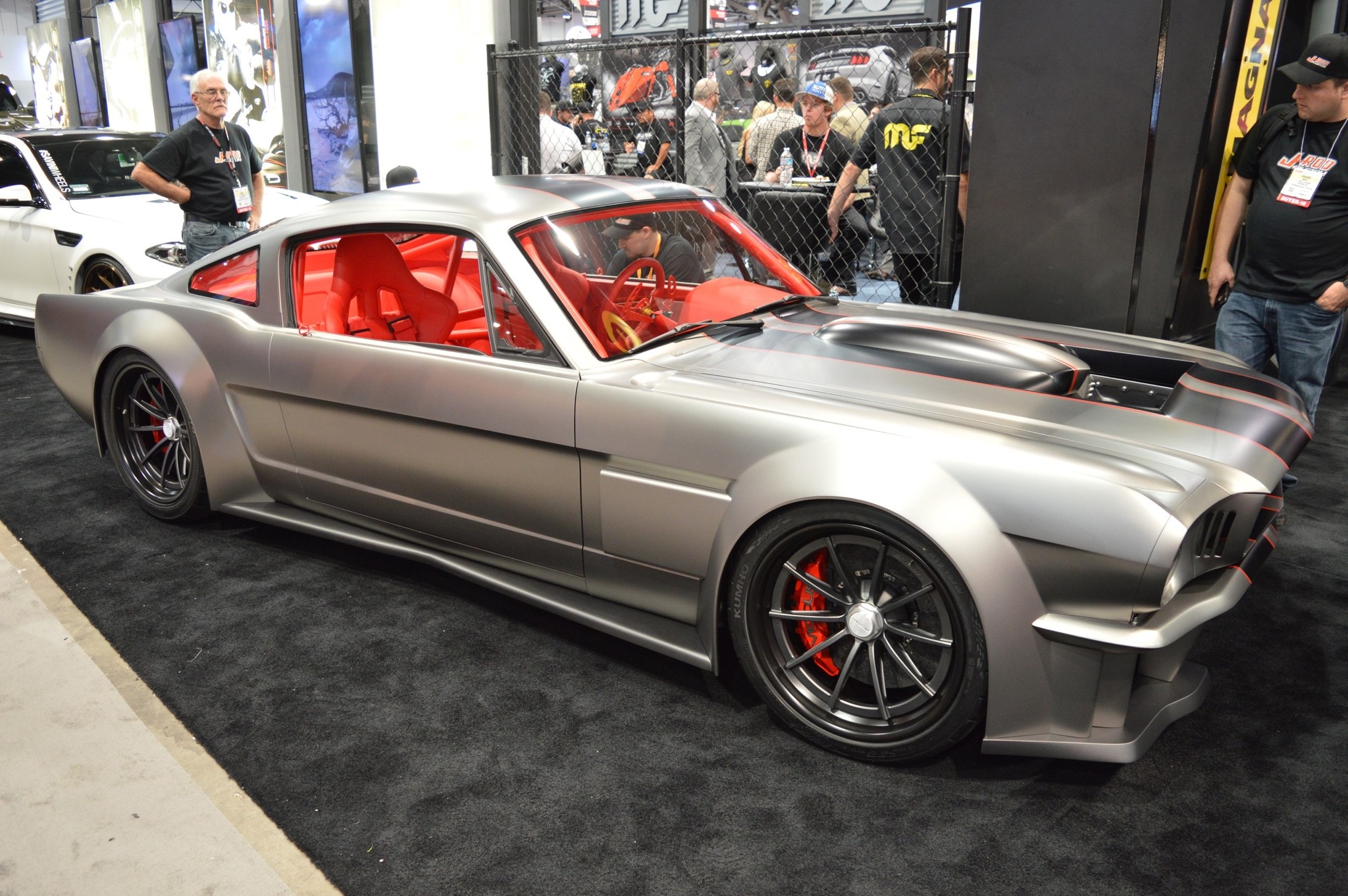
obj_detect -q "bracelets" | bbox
[1342,276,1348,288]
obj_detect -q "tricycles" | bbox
[606,59,676,117]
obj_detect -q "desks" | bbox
[739,180,876,293]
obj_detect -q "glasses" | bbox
[195,90,230,97]
[715,93,721,98]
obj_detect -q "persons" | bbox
[532,49,898,295]
[132,68,265,268]
[384,166,420,189]
[1202,31,1348,495]
[827,45,972,309]
[203,0,285,189]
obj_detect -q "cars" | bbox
[1,128,328,333]
[0,72,43,135]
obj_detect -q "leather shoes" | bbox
[831,286,856,296]
[865,269,897,281]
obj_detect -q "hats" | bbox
[796,82,833,105]
[577,101,592,113]
[1277,33,1348,85]
[601,213,656,240]
[717,44,734,65]
[760,49,775,66]
[633,100,653,111]
[557,100,574,110]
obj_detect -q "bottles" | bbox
[584,130,610,153]
[780,147,793,186]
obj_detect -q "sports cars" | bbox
[806,44,916,107]
[35,173,1316,767]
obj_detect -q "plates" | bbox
[792,177,831,183]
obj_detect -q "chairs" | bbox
[0,152,35,191]
[748,190,828,286]
[491,230,590,351]
[67,141,122,192]
[324,231,460,343]
